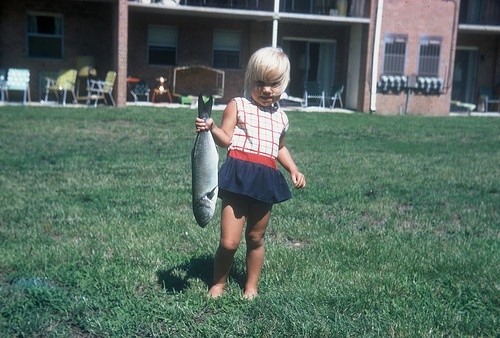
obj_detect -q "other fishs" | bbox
[191,95,219,229]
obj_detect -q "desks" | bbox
[128,77,140,101]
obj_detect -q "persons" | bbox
[196,46,306,301]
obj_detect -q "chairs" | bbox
[305,80,325,108]
[85,71,118,106]
[0,67,31,103]
[151,76,171,103]
[43,68,79,105]
[324,82,344,109]
